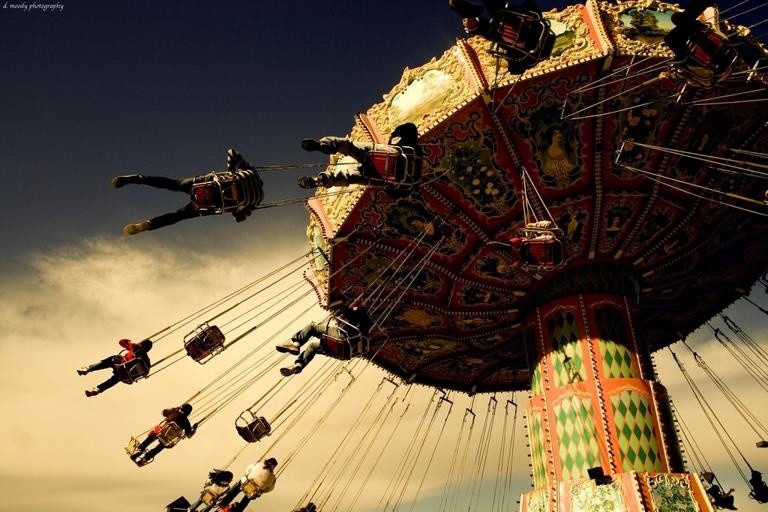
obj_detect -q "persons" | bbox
[662,1,732,100]
[111,146,263,237]
[76,335,156,398]
[448,1,556,76]
[297,120,422,201]
[708,484,738,510]
[218,457,278,511]
[129,402,199,466]
[187,468,233,512]
[748,469,768,504]
[276,302,376,376]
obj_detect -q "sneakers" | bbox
[280,364,301,376]
[297,175,329,189]
[301,139,332,155]
[276,343,300,355]
[111,174,143,189]
[130,451,142,460]
[137,457,147,466]
[76,367,89,376]
[123,219,152,236]
[85,390,98,396]
